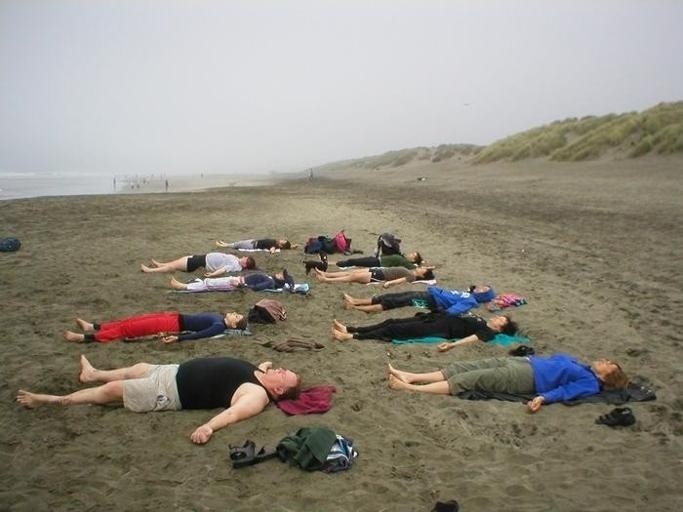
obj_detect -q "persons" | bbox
[215,238,291,254]
[140,251,255,277]
[328,314,518,351]
[170,269,294,291]
[343,285,496,316]
[16,353,301,444]
[327,249,436,269]
[314,266,435,289]
[386,352,628,413]
[62,311,247,344]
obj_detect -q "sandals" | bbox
[509,346,534,356]
[596,408,634,425]
[229,440,277,469]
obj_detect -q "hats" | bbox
[283,269,294,285]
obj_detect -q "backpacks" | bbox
[378,233,399,254]
